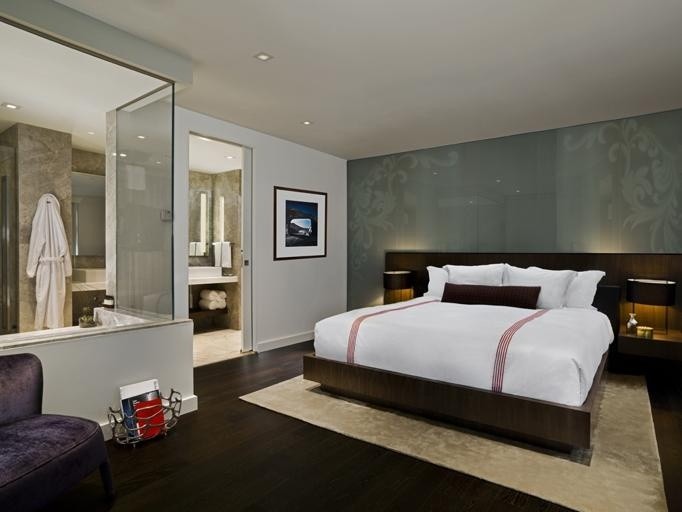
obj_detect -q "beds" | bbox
[304,284,619,455]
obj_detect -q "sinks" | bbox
[72,268,106,282]
[188,265,222,279]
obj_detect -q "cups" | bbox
[637,326,654,340]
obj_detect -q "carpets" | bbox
[239,373,669,512]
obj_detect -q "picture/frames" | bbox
[273,185,328,260]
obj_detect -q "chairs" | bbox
[0,353,113,512]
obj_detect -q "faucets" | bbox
[188,262,193,267]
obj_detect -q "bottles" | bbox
[626,312,638,335]
[103,295,114,309]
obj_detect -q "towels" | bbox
[196,242,205,256]
[198,289,227,310]
[214,242,221,266]
[190,242,196,257]
[221,242,232,268]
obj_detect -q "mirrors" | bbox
[70,171,212,257]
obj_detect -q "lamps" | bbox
[383,271,413,301]
[627,278,677,335]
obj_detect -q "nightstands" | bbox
[617,325,682,361]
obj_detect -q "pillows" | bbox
[424,263,606,310]
